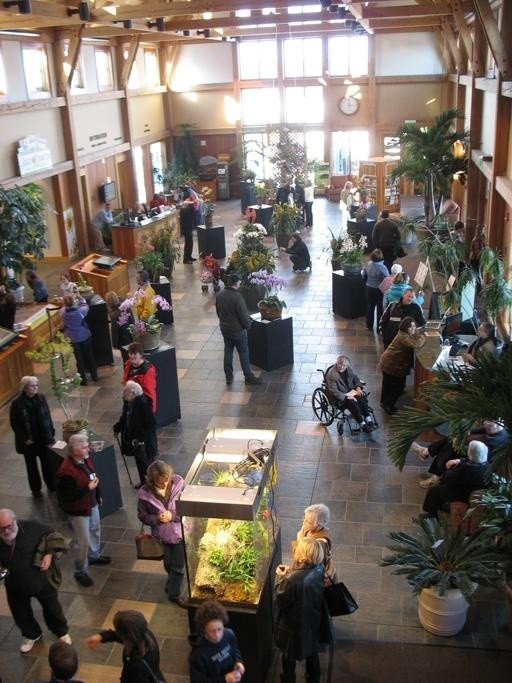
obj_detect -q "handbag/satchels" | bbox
[324,582,358,617]
[362,274,367,288]
[135,535,164,560]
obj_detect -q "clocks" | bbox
[338,96,358,116]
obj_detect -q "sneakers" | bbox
[420,477,441,488]
[226,379,233,384]
[362,421,377,432]
[74,572,93,586]
[32,484,55,497]
[175,594,190,608]
[60,634,72,645]
[184,258,196,264]
[309,261,312,270]
[20,634,42,653]
[135,483,142,488]
[295,269,304,273]
[245,377,264,384]
[80,376,98,385]
[410,442,429,461]
[380,402,397,415]
[89,556,111,565]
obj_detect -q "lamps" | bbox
[316,0,329,86]
[344,12,355,86]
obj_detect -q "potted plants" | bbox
[253,183,265,208]
[201,204,214,227]
[76,273,94,302]
[24,330,89,440]
[133,250,169,282]
[270,203,304,250]
[0,182,60,328]
[144,220,182,276]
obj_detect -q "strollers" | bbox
[196,251,225,294]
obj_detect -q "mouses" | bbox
[463,344,468,348]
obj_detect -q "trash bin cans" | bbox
[316,162,330,185]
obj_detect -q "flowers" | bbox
[118,280,171,332]
[223,222,287,290]
[339,233,368,263]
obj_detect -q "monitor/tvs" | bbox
[445,312,462,335]
[150,207,158,217]
[101,181,116,203]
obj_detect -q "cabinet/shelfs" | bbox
[359,157,401,213]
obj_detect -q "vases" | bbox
[340,258,364,275]
[243,289,265,312]
[257,293,287,319]
[132,325,161,352]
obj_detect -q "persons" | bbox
[326,176,511,521]
[1,178,315,411]
[0,376,336,682]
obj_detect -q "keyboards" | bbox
[450,344,462,356]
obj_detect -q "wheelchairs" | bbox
[312,364,382,437]
